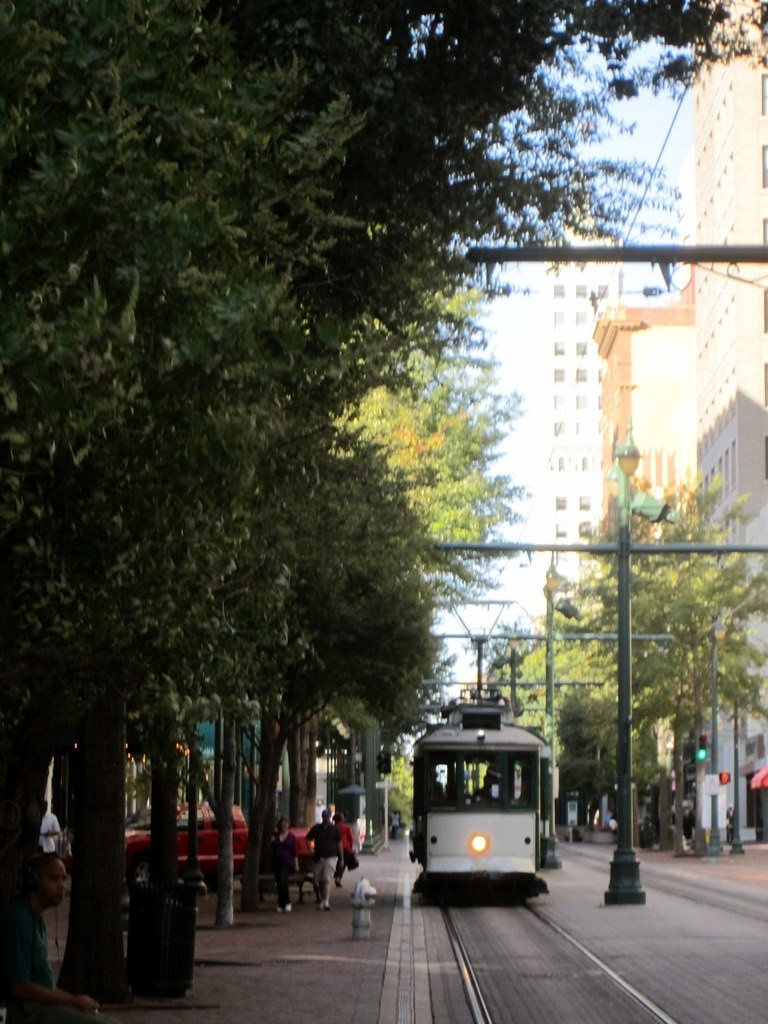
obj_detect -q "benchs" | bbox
[259,853,320,906]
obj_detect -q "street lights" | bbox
[606,431,680,913]
[702,621,724,860]
[537,554,577,871]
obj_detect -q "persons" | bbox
[609,816,617,834]
[314,799,336,824]
[726,809,734,842]
[305,809,344,911]
[0,853,100,1024]
[38,800,60,855]
[390,811,399,839]
[333,814,353,888]
[267,816,298,912]
[475,774,497,802]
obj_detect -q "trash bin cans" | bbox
[127,881,197,998]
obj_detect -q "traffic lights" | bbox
[378,750,392,774]
[718,772,732,785]
[696,733,708,761]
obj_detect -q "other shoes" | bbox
[276,904,285,913]
[316,899,330,910]
[334,879,342,888]
[284,903,292,912]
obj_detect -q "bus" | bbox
[410,638,552,902]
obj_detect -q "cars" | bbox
[124,803,249,889]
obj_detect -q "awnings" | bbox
[750,765,768,789]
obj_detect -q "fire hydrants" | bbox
[350,875,381,940]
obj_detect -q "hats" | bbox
[321,809,331,817]
